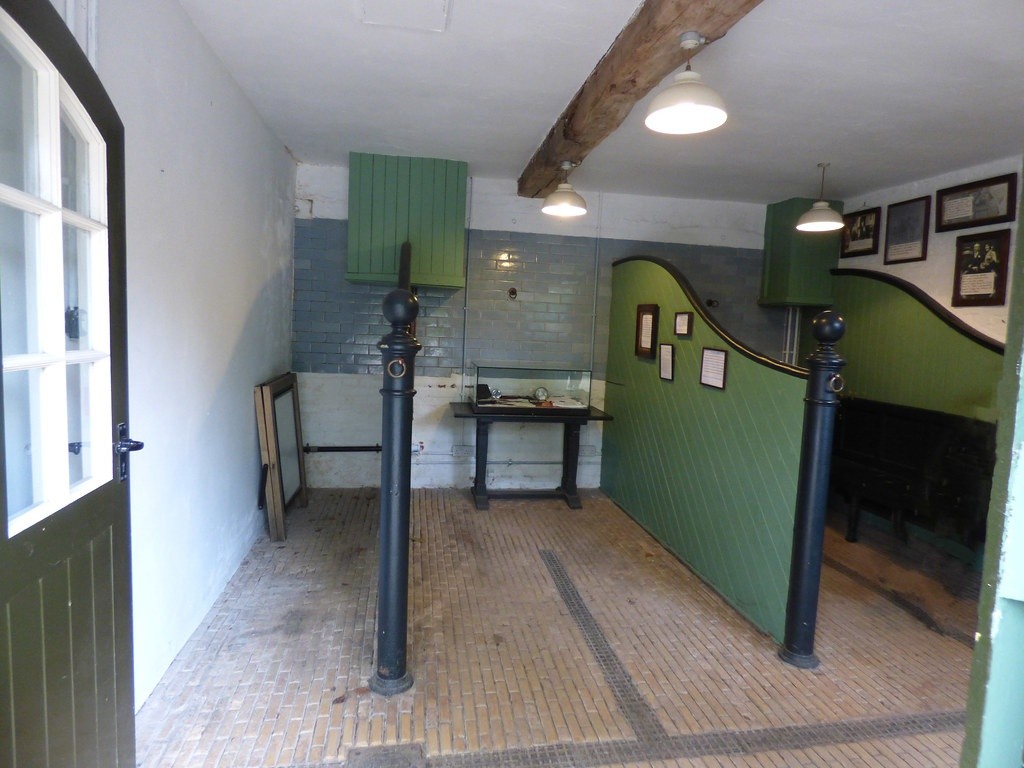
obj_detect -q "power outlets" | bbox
[453,445,475,457]
[579,445,596,456]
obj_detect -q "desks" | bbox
[451,402,614,510]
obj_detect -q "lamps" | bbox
[795,163,845,232]
[541,161,587,216]
[645,59,727,134]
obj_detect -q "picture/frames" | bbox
[951,229,1011,307]
[935,173,1017,233]
[839,203,881,259]
[700,347,728,389]
[674,312,693,336]
[635,304,659,359]
[883,196,931,265]
[659,344,674,381]
